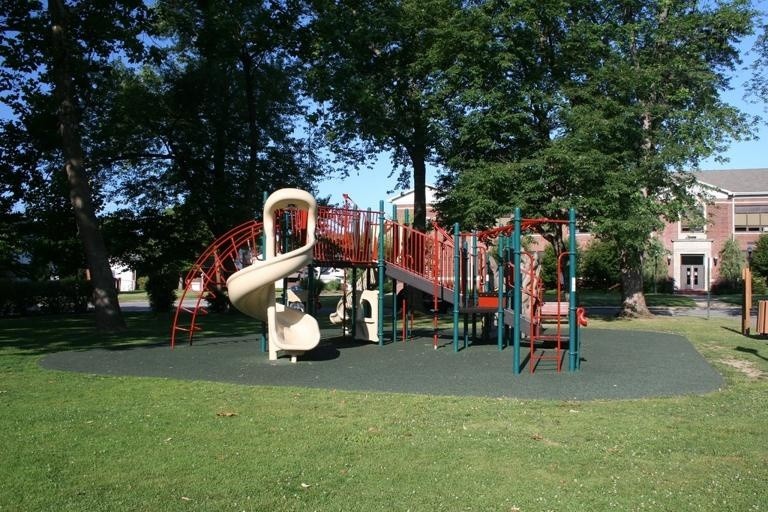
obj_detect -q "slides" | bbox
[225,188,321,361]
[329,268,388,326]
